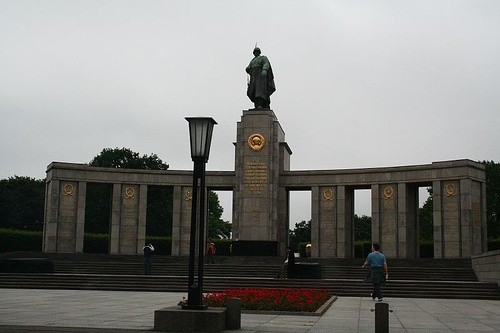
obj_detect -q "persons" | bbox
[361,242,388,302]
[280,247,295,280]
[246,47,276,110]
[206,238,216,264]
[142,242,155,274]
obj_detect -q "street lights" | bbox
[181,116,218,310]
